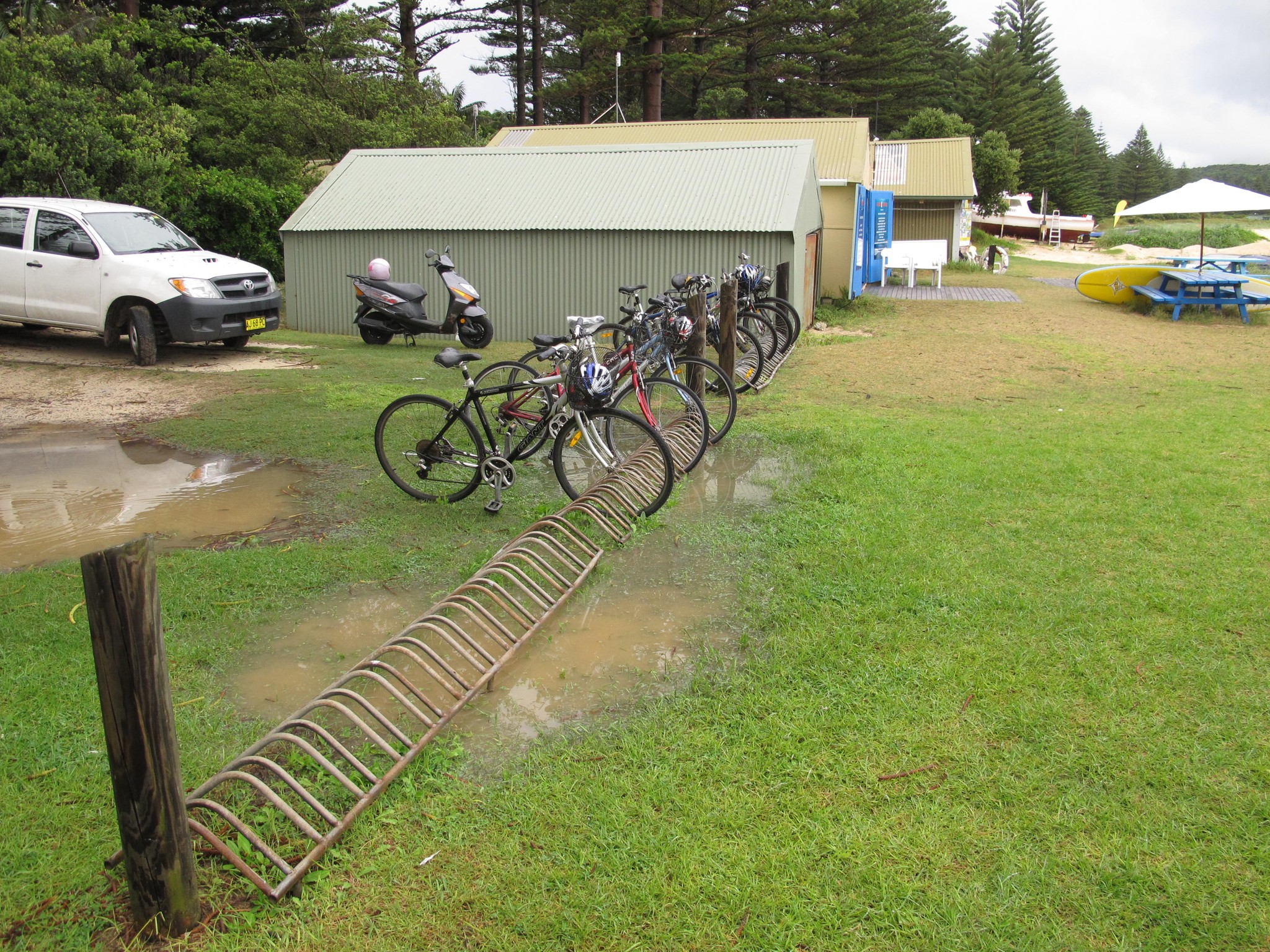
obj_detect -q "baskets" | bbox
[735,264,778,296]
[661,315,700,349]
[737,296,750,316]
[633,324,677,364]
[566,346,623,412]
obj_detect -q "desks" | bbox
[1151,254,1267,276]
[1130,267,1270,324]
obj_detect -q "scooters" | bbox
[347,245,494,350]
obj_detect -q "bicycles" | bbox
[375,254,801,521]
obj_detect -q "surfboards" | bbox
[1075,265,1270,314]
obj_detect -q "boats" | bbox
[972,192,1094,243]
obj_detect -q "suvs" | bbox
[0,197,281,366]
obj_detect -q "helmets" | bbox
[739,264,758,291]
[580,362,612,407]
[665,316,693,345]
[368,258,391,280]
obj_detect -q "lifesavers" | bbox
[978,245,1009,277]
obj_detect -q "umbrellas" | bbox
[1112,177,1270,316]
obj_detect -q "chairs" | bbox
[880,247,944,289]
